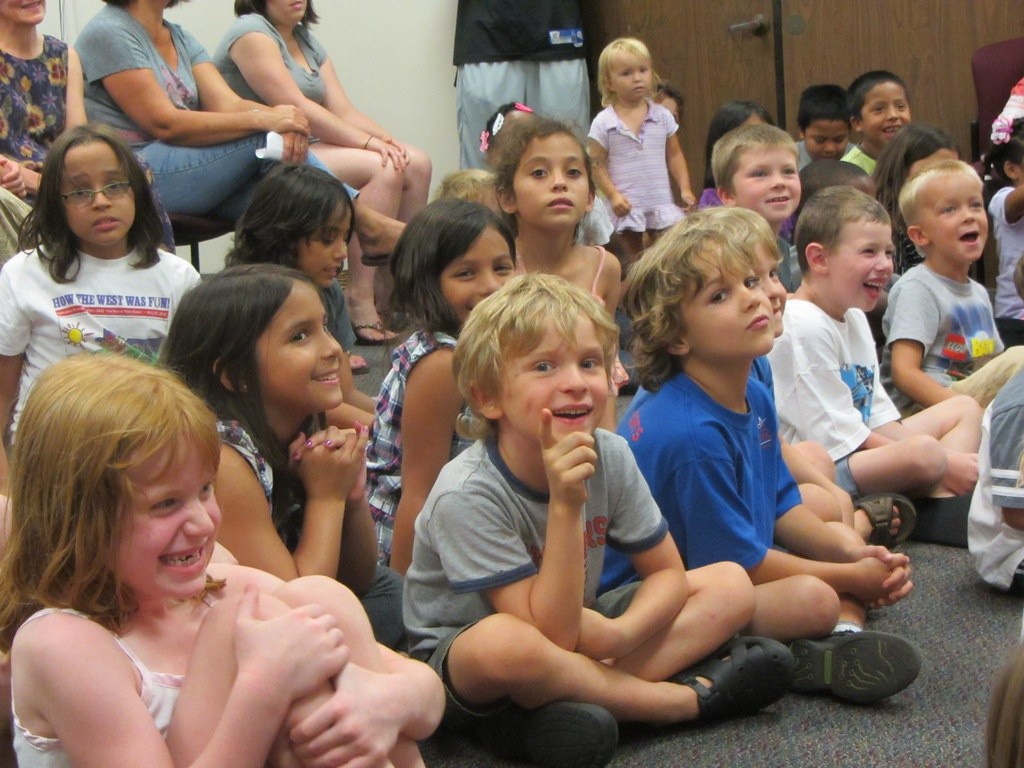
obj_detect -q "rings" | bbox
[300,144,306,150]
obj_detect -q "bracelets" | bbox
[365,136,373,150]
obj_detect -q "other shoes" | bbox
[917,497,975,548]
[350,365,370,374]
[349,320,398,345]
[487,703,617,768]
[788,629,921,705]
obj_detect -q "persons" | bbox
[594,226,924,707]
[0,350,447,768]
[0,0,1024,768]
[397,271,798,768]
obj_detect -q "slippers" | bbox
[361,254,392,267]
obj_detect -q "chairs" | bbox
[970,35,1024,186]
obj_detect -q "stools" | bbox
[155,213,234,273]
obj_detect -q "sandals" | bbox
[852,492,916,549]
[671,636,796,722]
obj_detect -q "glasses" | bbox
[56,181,130,209]
